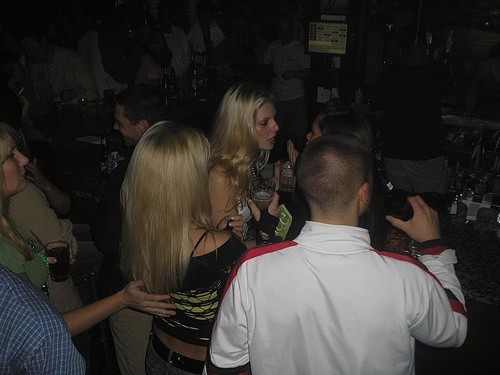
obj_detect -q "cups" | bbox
[250,177,276,212]
[279,160,296,192]
[95,98,105,117]
[45,240,71,282]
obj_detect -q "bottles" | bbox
[99,136,110,174]
[162,66,169,90]
[191,69,199,98]
[379,174,414,221]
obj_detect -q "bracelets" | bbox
[418,238,447,250]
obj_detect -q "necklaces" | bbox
[9,224,35,250]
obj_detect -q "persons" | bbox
[202,129,467,375]
[204,84,293,250]
[285,99,382,249]
[119,118,282,375]
[91,81,176,299]
[0,264,91,375]
[0,123,175,338]
[0,0,500,219]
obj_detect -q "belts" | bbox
[149,332,205,375]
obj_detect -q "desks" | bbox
[372,212,500,304]
